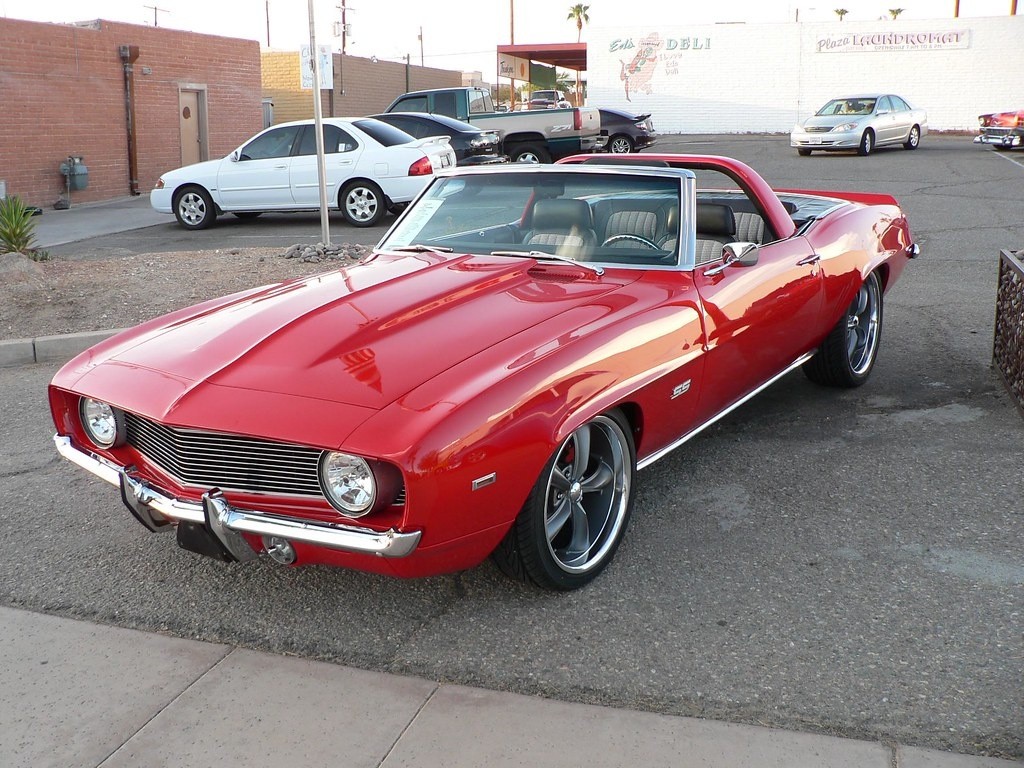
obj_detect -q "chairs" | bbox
[651,204,741,265]
[522,199,598,248]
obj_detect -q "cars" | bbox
[598,107,658,155]
[367,112,506,166]
[972,109,1024,150]
[150,116,457,231]
[790,92,928,157]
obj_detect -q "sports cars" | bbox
[46,152,920,595]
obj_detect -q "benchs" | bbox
[595,199,774,250]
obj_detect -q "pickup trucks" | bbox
[386,85,610,165]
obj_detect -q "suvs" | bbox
[494,89,571,114]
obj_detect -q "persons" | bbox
[837,102,855,114]
